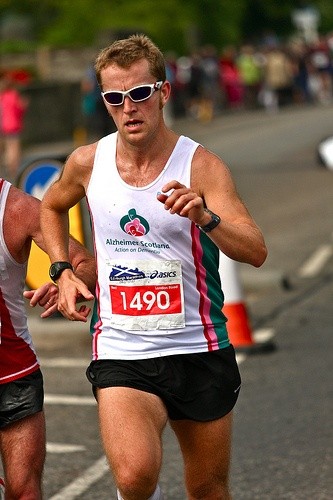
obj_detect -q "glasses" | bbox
[101,81,163,106]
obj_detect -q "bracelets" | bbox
[195,208,221,233]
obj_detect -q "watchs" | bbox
[49,261,73,283]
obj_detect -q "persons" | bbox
[39,32,269,500]
[0,177,97,500]
[0,30,333,175]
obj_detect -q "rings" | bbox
[47,302,51,306]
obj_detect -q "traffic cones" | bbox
[217,250,276,355]
